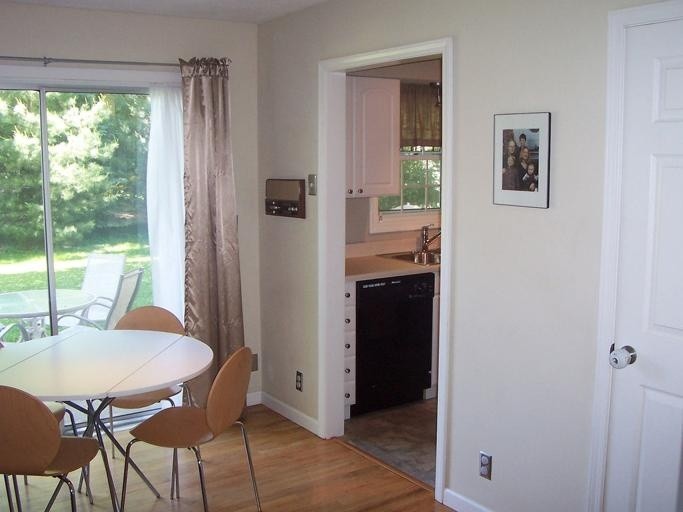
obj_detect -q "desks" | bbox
[0,289,97,338]
[1,328,214,495]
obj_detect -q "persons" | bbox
[502,132,537,191]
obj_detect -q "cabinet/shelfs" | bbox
[345,278,357,421]
[338,73,401,200]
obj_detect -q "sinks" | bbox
[392,253,441,263]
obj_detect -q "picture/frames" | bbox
[490,110,553,209]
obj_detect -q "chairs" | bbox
[44,252,125,335]
[55,271,143,330]
[0,321,28,346]
[121,347,260,512]
[0,386,115,512]
[79,305,190,499]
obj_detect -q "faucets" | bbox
[422,224,441,249]
